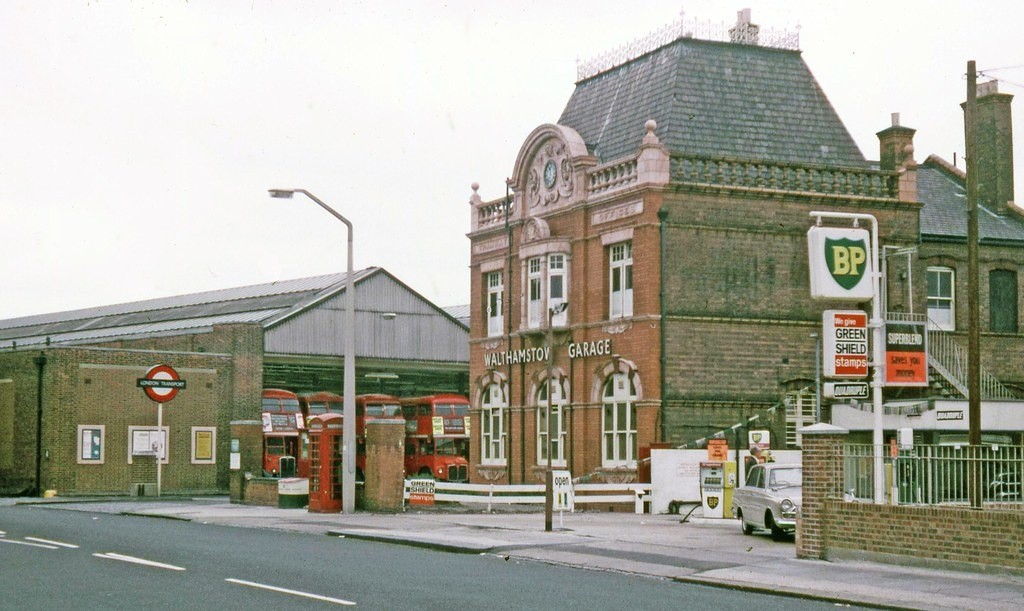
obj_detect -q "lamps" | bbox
[901,271,907,280]
[379,313,397,320]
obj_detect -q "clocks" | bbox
[543,158,557,189]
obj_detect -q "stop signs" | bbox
[137,364,187,402]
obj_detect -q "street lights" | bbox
[267,187,356,516]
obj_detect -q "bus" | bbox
[296,391,343,430]
[399,393,470,484]
[355,392,406,482]
[261,388,306,478]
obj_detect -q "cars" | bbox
[732,461,861,542]
